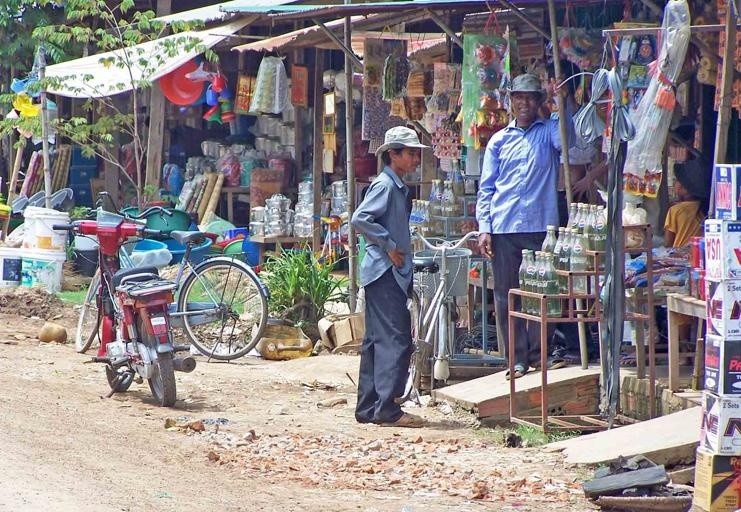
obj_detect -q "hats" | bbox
[672,159,708,199]
[505,73,548,102]
[669,123,696,149]
[374,126,429,157]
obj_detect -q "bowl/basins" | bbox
[75,201,273,275]
[160,56,210,106]
[9,188,76,214]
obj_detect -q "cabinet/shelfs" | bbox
[182,143,299,230]
[666,166,711,401]
[507,200,661,432]
[249,178,353,271]
[410,158,508,369]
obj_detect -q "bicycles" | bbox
[394,227,480,406]
[75,190,271,364]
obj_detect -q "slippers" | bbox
[380,412,428,428]
[505,364,525,380]
[535,358,566,370]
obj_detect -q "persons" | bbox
[476,74,575,379]
[548,60,610,366]
[351,127,432,427]
[620,158,711,366]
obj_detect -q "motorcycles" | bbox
[52,211,196,407]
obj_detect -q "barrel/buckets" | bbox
[24,207,69,253]
[0,247,23,286]
[0,204,10,224]
[22,248,66,292]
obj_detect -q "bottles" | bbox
[573,203,582,229]
[586,204,597,266]
[544,226,557,253]
[595,204,607,269]
[564,229,571,293]
[522,247,535,314]
[440,180,447,236]
[553,227,563,269]
[411,199,417,213]
[579,204,587,234]
[416,200,420,211]
[543,252,563,316]
[432,180,442,235]
[535,253,544,316]
[444,181,458,236]
[419,200,424,233]
[567,202,576,227]
[423,201,432,237]
[543,225,550,249]
[571,227,588,293]
[518,249,528,312]
[452,158,466,195]
[429,180,435,202]
